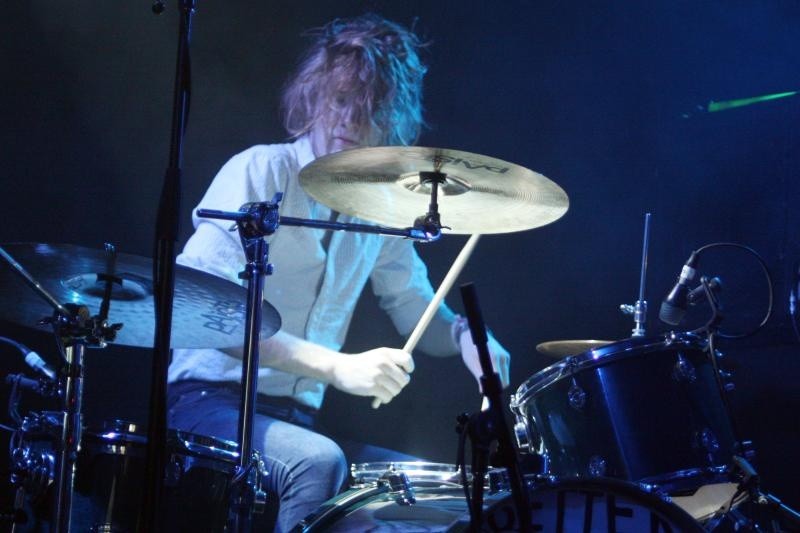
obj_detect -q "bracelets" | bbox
[457,318,490,340]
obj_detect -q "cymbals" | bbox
[536,340,616,358]
[297,144,571,235]
[1,240,283,349]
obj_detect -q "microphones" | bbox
[18,343,57,385]
[660,251,701,325]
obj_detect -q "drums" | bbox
[657,483,782,533]
[509,332,740,481]
[11,411,259,533]
[284,461,703,533]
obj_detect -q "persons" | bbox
[165,15,508,533]
